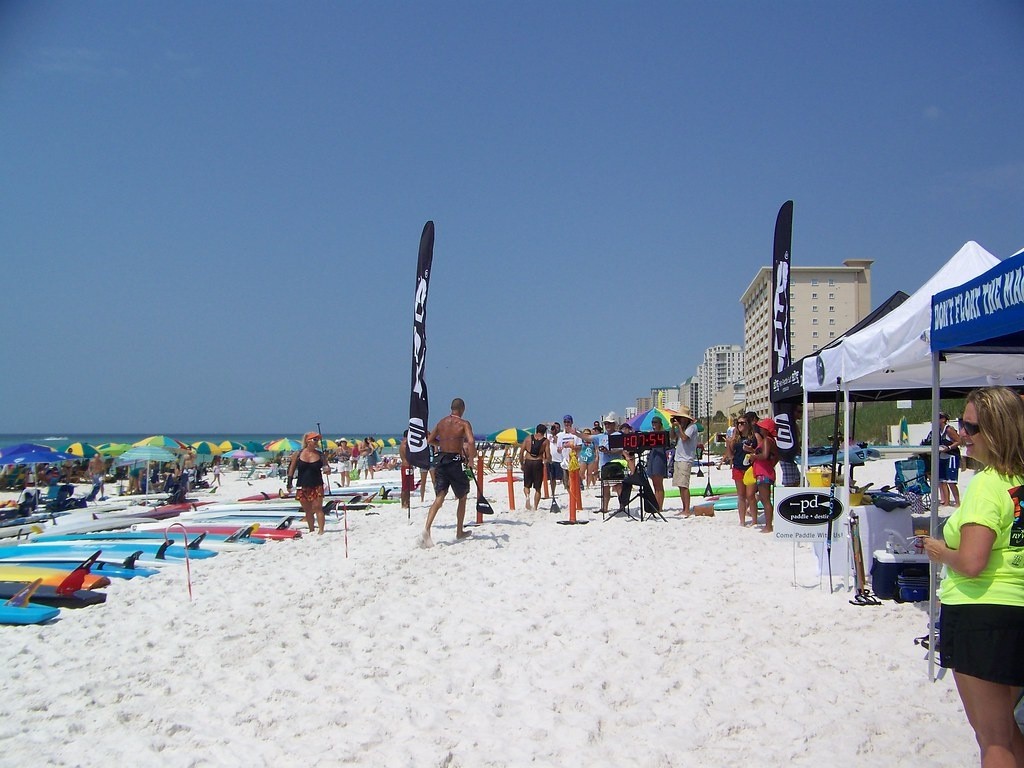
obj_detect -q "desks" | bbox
[849,505,913,583]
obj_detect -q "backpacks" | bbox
[525,434,547,457]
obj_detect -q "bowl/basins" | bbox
[850,493,864,505]
[806,472,832,487]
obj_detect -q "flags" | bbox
[771,200,794,379]
[405,221,435,469]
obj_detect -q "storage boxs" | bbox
[869,550,930,600]
[805,473,832,487]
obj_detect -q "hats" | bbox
[601,416,616,422]
[756,419,778,437]
[306,433,322,441]
[671,405,694,421]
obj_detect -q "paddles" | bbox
[462,447,496,515]
[548,462,561,513]
[317,422,332,495]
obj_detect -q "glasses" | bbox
[308,439,318,441]
[737,421,747,426]
[958,418,980,437]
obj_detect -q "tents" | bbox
[769,241,1024,683]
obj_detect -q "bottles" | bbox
[885,532,894,553]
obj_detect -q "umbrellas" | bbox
[319,438,401,455]
[486,428,529,444]
[0,435,302,513]
[899,416,908,446]
[626,407,704,432]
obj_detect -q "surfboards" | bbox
[867,444,945,453]
[663,484,774,497]
[1,486,423,624]
[689,495,775,510]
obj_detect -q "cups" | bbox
[914,530,928,554]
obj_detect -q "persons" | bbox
[519,405,699,517]
[779,403,810,487]
[717,412,779,533]
[3,447,226,518]
[924,386,1024,768]
[927,412,962,507]
[286,432,331,535]
[421,431,435,502]
[423,398,477,548]
[335,437,378,487]
[399,430,415,508]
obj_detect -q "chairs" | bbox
[0,482,101,522]
[162,469,201,493]
[894,459,931,511]
[473,444,520,475]
